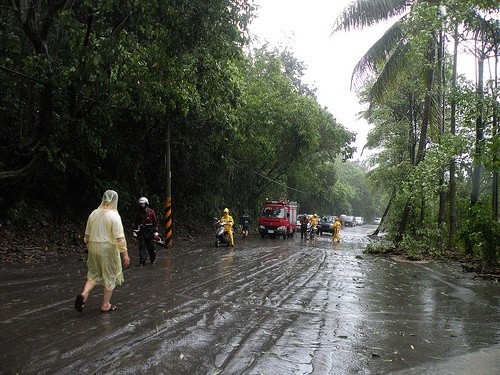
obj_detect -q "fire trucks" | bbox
[258,198,297,239]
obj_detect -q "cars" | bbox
[297,215,338,235]
[340,214,367,227]
[373,218,381,225]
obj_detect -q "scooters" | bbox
[305,225,318,240]
[214,217,235,247]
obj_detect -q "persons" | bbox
[215,208,234,247]
[132,197,159,268]
[75,190,130,313]
[240,212,251,238]
[300,213,341,243]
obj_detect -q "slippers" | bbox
[101,305,117,311]
[75,295,85,312]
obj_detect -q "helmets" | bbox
[138,197,149,204]
[313,214,317,217]
